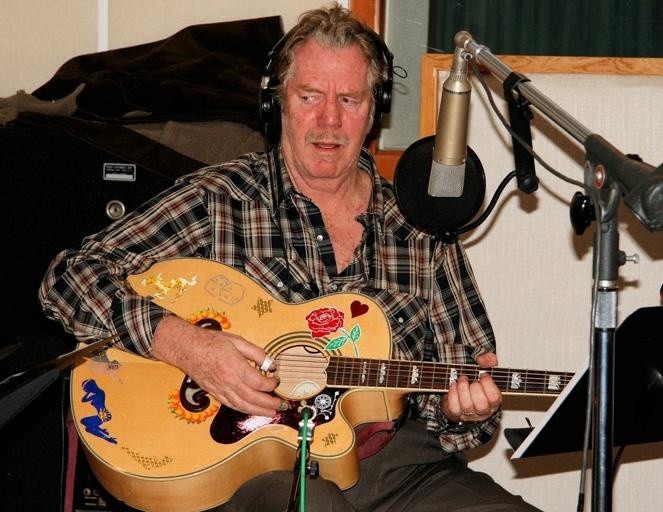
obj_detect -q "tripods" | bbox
[475,45,662,511]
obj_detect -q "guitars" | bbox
[67,255,574,512]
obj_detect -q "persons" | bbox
[38,4,543,512]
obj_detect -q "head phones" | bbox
[257,19,397,152]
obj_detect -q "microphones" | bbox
[426,30,474,198]
